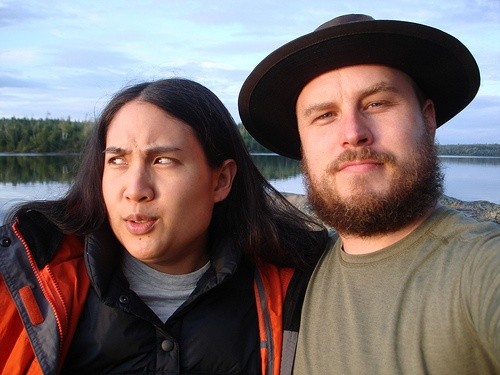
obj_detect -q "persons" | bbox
[0,77,330,375]
[238,13,500,375]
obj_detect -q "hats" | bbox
[238,14,481,159]
